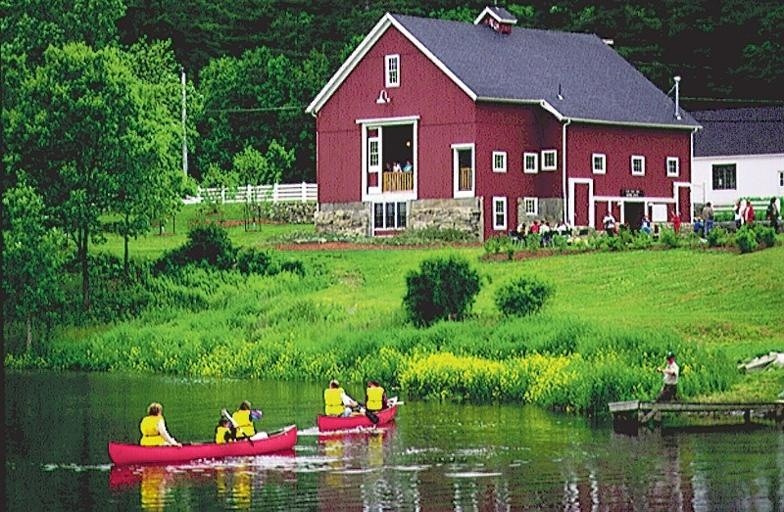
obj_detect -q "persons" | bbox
[732,199,754,230]
[657,353,679,403]
[214,401,263,444]
[693,202,714,237]
[516,221,574,248]
[139,402,184,447]
[671,210,681,235]
[602,212,660,241]
[324,378,358,417]
[385,159,413,173]
[364,381,387,413]
[765,196,780,234]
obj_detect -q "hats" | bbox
[665,351,674,359]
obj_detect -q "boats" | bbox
[108,424,297,467]
[317,404,397,432]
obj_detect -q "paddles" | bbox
[350,399,379,423]
[225,411,255,446]
